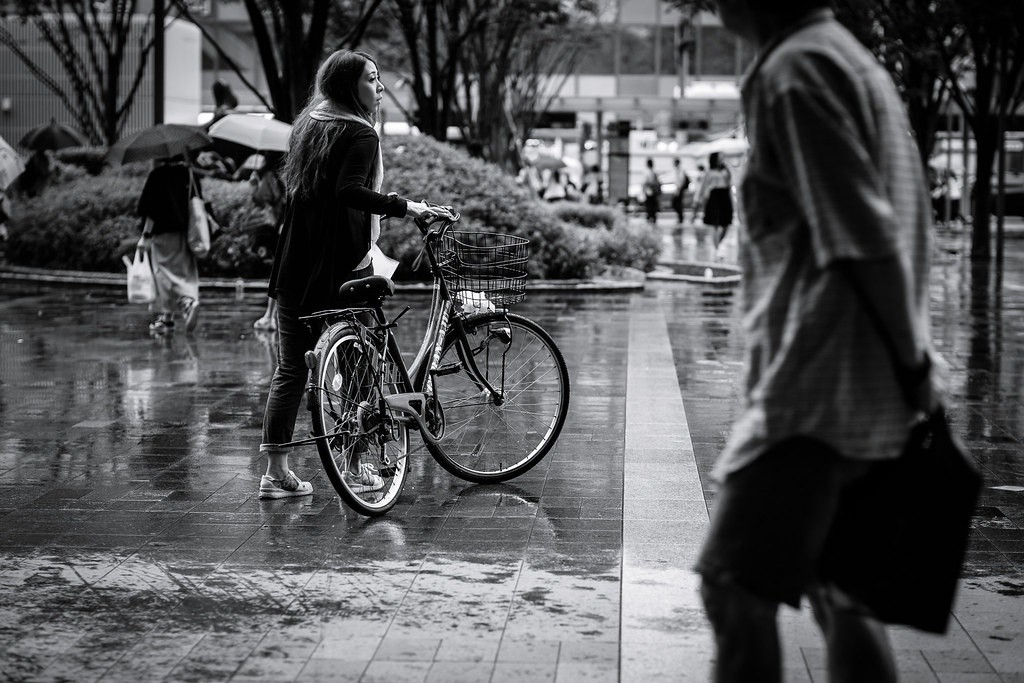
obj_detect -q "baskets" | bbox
[432,229,529,314]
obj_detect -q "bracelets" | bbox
[141,231,154,239]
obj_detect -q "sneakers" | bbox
[342,462,385,493]
[258,468,314,498]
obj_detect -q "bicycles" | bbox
[278,193,572,517]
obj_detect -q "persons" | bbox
[260,48,451,502]
[579,166,604,204]
[139,154,204,334]
[691,153,733,264]
[518,159,543,197]
[694,1,950,682]
[643,158,663,222]
[542,168,570,202]
[6,148,49,201]
[670,159,687,228]
[255,152,289,330]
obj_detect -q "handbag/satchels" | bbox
[121,247,156,305]
[847,402,983,633]
[186,165,210,257]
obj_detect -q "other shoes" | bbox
[149,316,175,334]
[253,317,277,330]
[178,295,200,331]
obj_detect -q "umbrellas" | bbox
[18,113,85,155]
[212,113,294,153]
[532,154,566,169]
[0,134,26,191]
[111,123,213,167]
